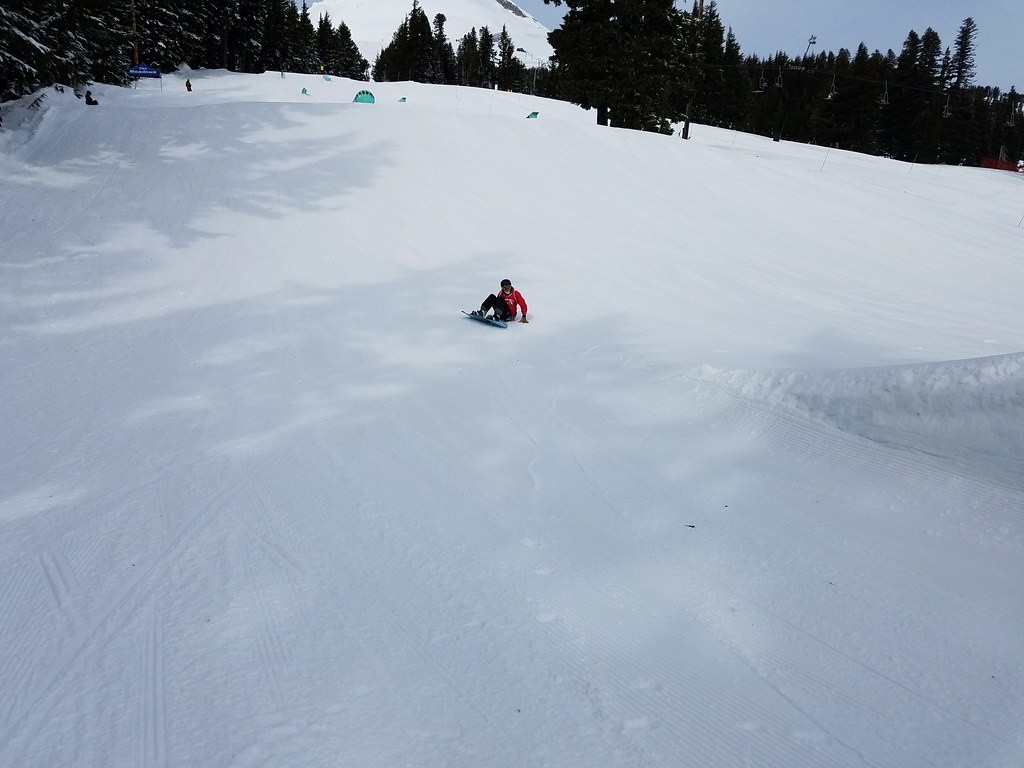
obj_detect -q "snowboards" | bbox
[461,310,509,329]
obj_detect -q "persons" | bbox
[85,91,98,105]
[472,279,528,323]
[186,79,192,91]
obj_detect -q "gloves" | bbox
[519,317,529,323]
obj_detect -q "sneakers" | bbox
[486,314,500,322]
[472,310,486,317]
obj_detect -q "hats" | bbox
[500,279,511,287]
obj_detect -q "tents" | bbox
[126,63,162,90]
